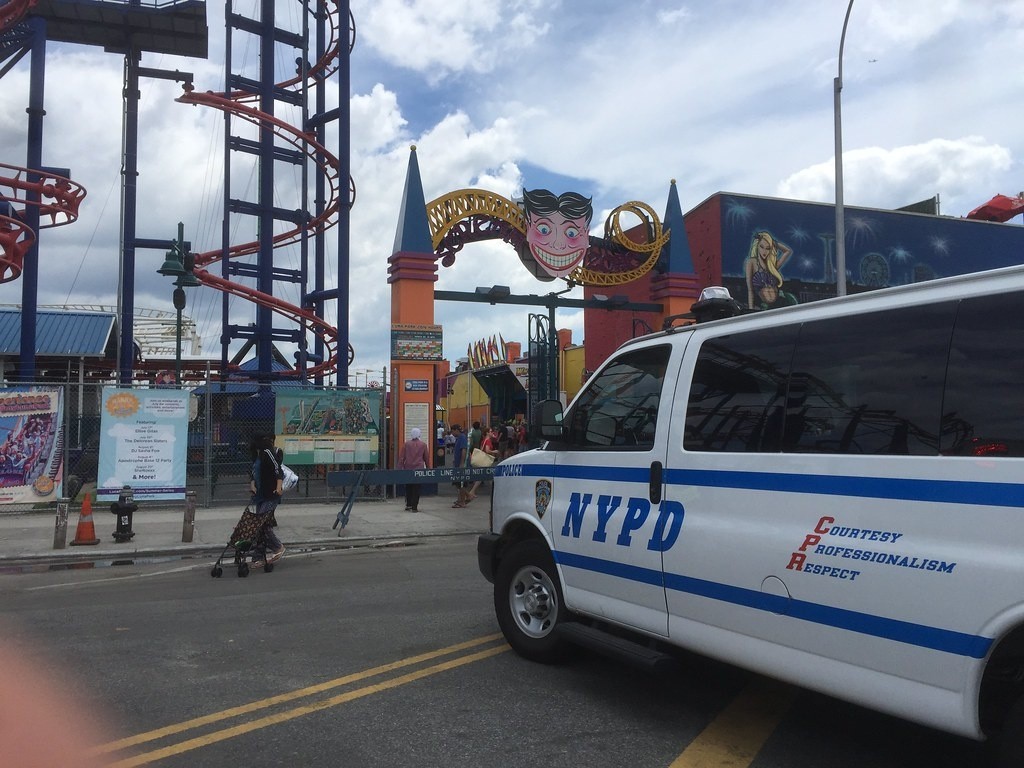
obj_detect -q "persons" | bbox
[437,423,456,448]
[450,424,475,508]
[266,431,283,527]
[470,427,498,498]
[471,421,527,463]
[397,428,430,513]
[251,433,286,569]
[0,419,46,470]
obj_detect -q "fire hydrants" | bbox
[111,485,139,543]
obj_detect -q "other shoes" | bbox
[405,506,412,510]
[452,501,466,508]
[412,509,418,512]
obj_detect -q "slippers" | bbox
[249,560,266,569]
[269,547,286,566]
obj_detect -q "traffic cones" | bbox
[69,492,100,545]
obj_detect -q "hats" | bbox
[451,424,462,430]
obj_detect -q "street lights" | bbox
[156,221,201,388]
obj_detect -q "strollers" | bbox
[211,491,273,579]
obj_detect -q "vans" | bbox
[477,263,1023,756]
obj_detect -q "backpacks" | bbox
[264,447,300,498]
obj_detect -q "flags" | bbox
[467,336,508,369]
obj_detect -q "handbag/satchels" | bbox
[470,438,496,468]
